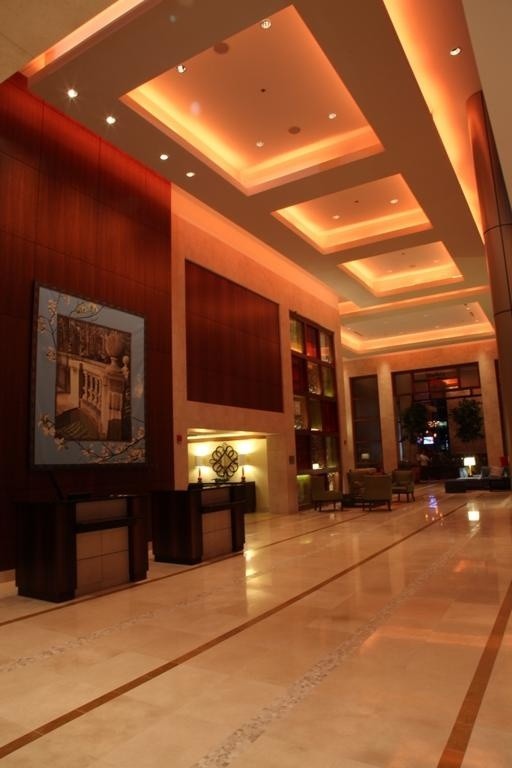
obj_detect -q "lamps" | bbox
[464,456,476,477]
[361,452,371,462]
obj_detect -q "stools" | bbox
[444,467,511,493]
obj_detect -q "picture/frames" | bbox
[27,278,151,473]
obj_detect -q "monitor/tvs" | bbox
[423,435,435,446]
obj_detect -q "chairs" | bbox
[309,468,415,511]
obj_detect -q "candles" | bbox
[199,467,201,478]
[242,467,244,476]
